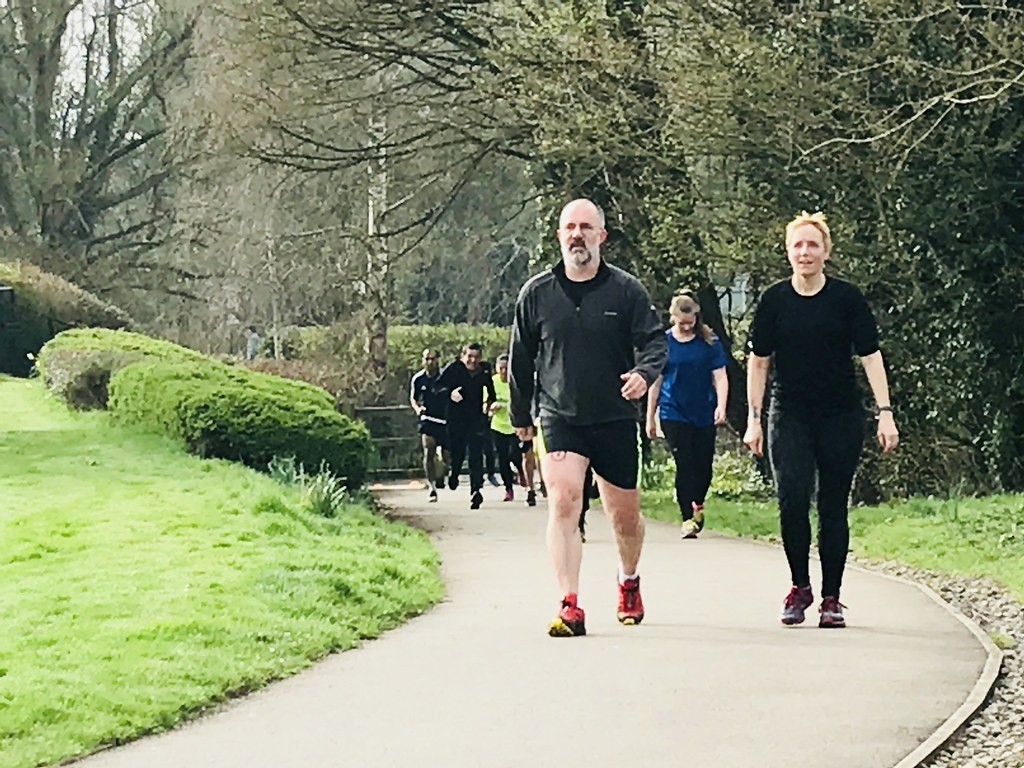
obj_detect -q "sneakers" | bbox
[549,594,586,637]
[782,585,814,625]
[692,501,705,533]
[617,568,644,625]
[682,518,699,538]
[820,597,847,628]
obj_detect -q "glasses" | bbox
[425,357,435,361]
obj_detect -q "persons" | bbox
[577,468,598,543]
[410,342,547,510]
[509,198,674,639]
[743,209,902,630]
[243,325,261,360]
[646,293,729,539]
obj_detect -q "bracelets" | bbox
[877,406,893,415]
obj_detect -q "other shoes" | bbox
[520,478,526,486]
[469,491,483,509]
[504,491,513,501]
[527,490,536,506]
[579,514,587,543]
[429,491,437,501]
[540,481,547,497]
[488,476,500,486]
[436,478,445,488]
[448,470,459,490]
